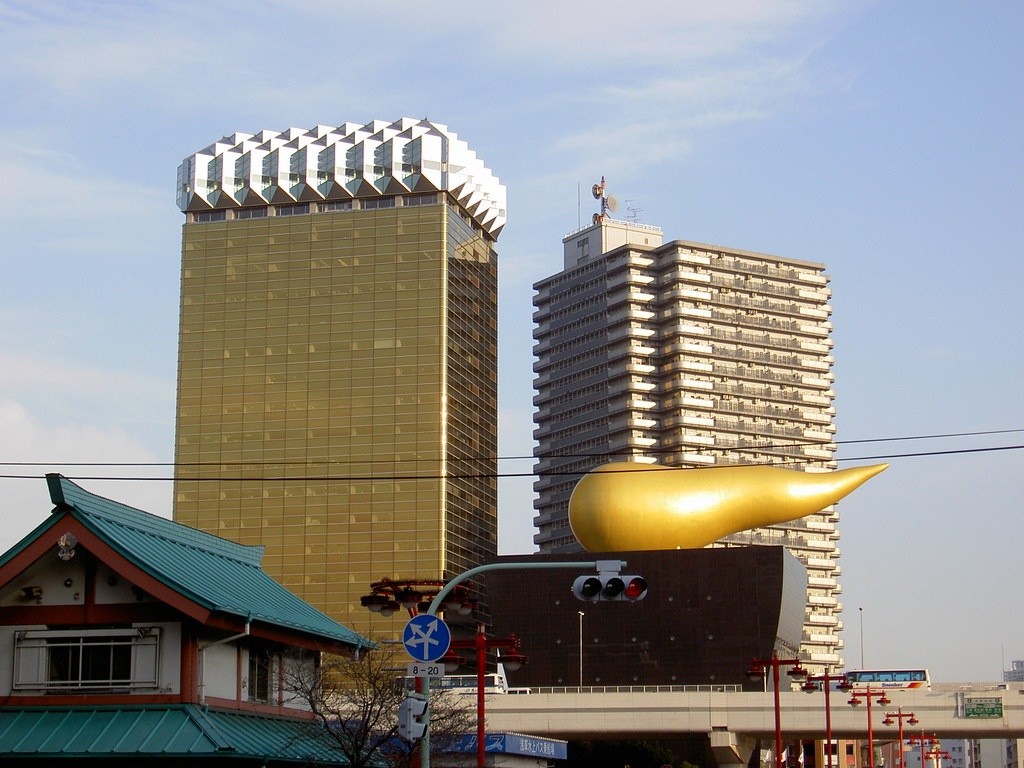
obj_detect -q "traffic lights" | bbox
[572,574,649,604]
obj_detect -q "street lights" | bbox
[434,627,533,768]
[745,654,808,768]
[846,685,892,768]
[881,705,918,768]
[360,575,481,768]
[907,729,940,768]
[925,746,952,768]
[802,672,854,768]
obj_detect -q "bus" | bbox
[844,669,932,692]
